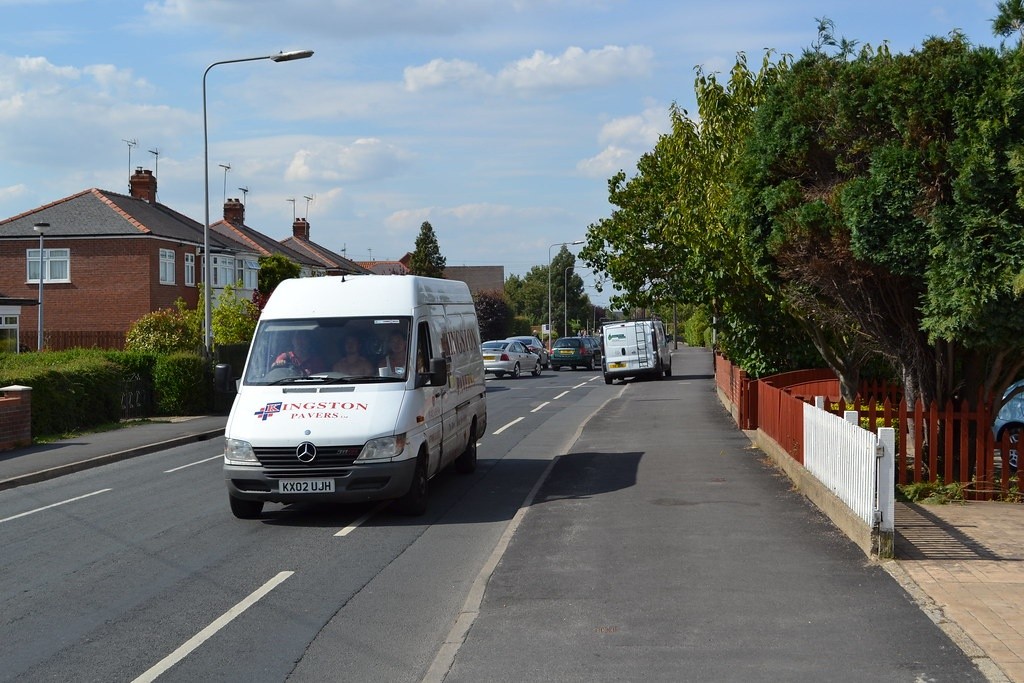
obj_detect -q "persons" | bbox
[330,333,374,376]
[275,330,325,377]
[380,331,424,377]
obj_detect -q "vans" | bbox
[218,242,490,519]
[549,334,603,371]
[602,318,673,384]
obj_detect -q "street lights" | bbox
[548,239,585,354]
[564,266,591,336]
[200,48,320,364]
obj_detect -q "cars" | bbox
[506,336,548,370]
[478,339,542,381]
